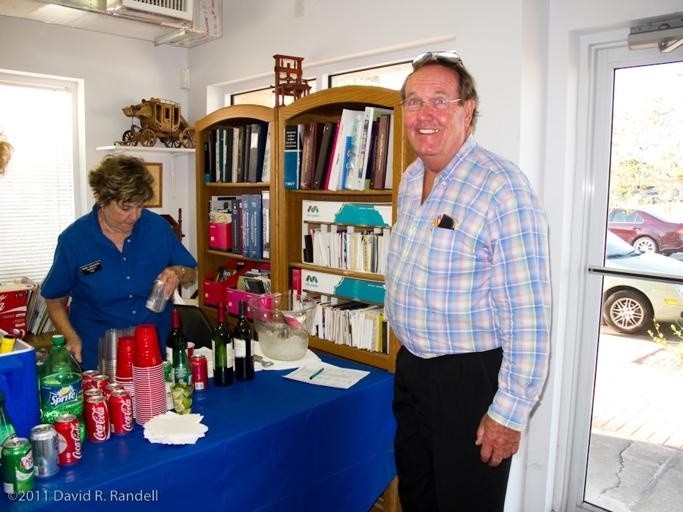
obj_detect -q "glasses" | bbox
[398,96,468,111]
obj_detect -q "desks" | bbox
[0,346,398,512]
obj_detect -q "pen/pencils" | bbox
[309,368,324,379]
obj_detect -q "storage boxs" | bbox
[0,328,41,442]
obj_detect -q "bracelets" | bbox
[177,266,186,281]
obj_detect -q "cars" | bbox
[606,207,683,257]
[600,229,683,335]
[608,160,682,206]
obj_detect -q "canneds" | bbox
[53,414,83,464]
[163,361,172,382]
[2,437,35,495]
[81,370,133,443]
[190,355,209,390]
[144,280,167,314]
[30,424,58,477]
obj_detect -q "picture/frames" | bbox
[138,161,163,208]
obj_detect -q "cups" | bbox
[98,322,168,427]
[188,341,194,360]
[169,381,194,417]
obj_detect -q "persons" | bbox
[40,153,199,373]
[384,49,553,512]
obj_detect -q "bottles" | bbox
[40,334,85,444]
[233,300,254,381]
[0,390,16,475]
[166,308,188,363]
[172,338,192,384]
[211,302,234,387]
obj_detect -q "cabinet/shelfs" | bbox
[276,88,417,375]
[196,104,275,324]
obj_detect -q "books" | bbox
[22,276,72,336]
